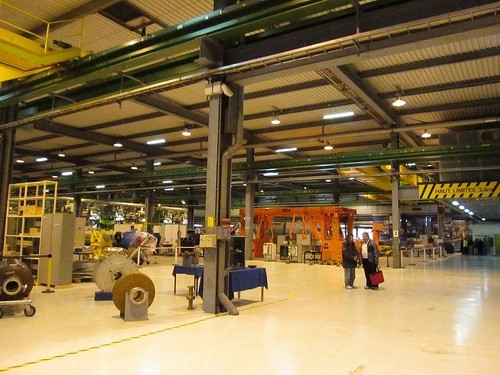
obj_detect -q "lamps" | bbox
[392,86,406,108]
[181,121,192,136]
[270,105,282,124]
[421,129,431,138]
[323,140,333,150]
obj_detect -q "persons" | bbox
[341,233,360,289]
[180,237,199,256]
[357,232,379,290]
[460,235,487,256]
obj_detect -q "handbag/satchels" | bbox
[369,264,384,285]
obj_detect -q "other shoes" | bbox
[364,286,379,290]
[352,285,358,289]
[345,285,352,289]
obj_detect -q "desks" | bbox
[303,250,322,263]
[172,263,268,301]
